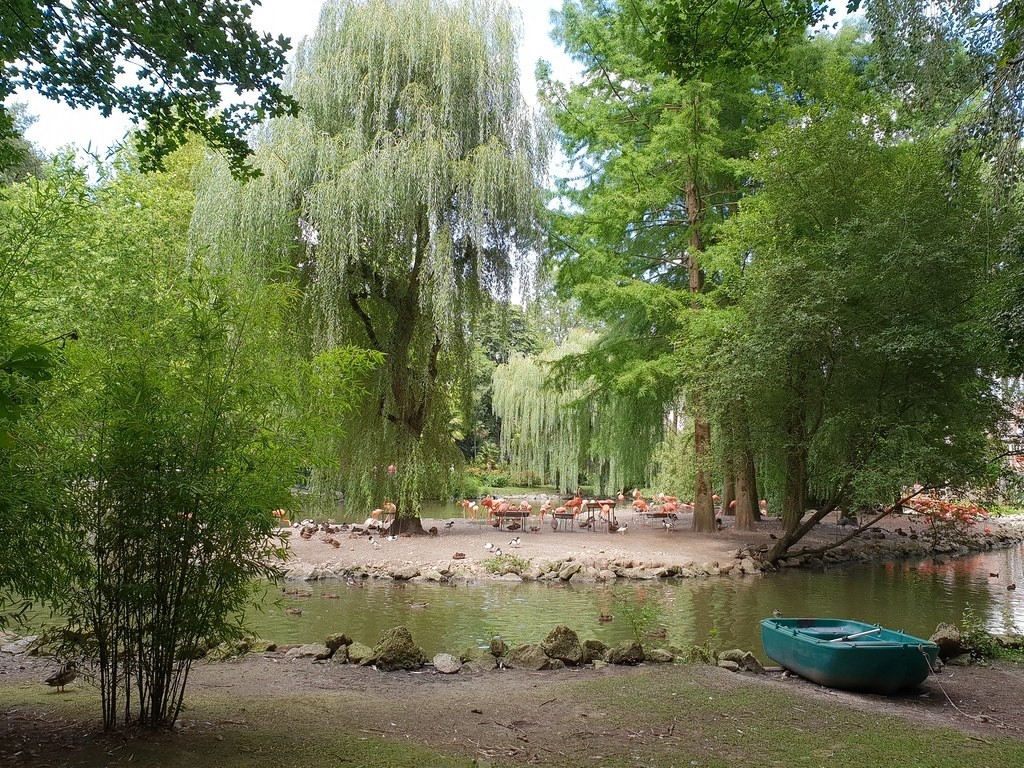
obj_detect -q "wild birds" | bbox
[269,464,1017,640]
[39,661,78,692]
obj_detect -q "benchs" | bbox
[797,627,860,637]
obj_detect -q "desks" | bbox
[555,513,574,532]
[645,512,672,530]
[381,511,398,535]
[493,511,530,532]
[583,499,616,531]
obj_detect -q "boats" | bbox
[760,616,941,695]
